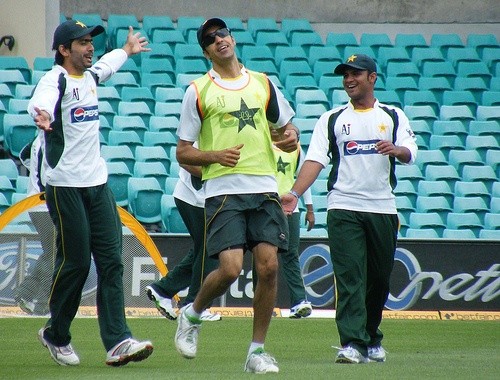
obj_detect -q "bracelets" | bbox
[290,128,299,142]
[288,190,302,198]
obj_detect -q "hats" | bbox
[197,17,227,47]
[335,54,377,78]
[54,20,104,48]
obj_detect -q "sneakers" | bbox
[175,303,201,357]
[198,310,222,321]
[143,286,177,320]
[37,327,80,366]
[331,345,370,364]
[243,347,279,374]
[368,346,388,362]
[106,337,154,366]
[290,301,312,318]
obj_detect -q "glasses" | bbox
[202,28,229,48]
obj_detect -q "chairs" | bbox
[0,13,500,240]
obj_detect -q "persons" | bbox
[16,18,158,366]
[176,19,301,375]
[253,107,320,320]
[146,137,246,324]
[271,52,417,365]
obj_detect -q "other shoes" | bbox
[14,294,34,314]
[34,303,50,315]
